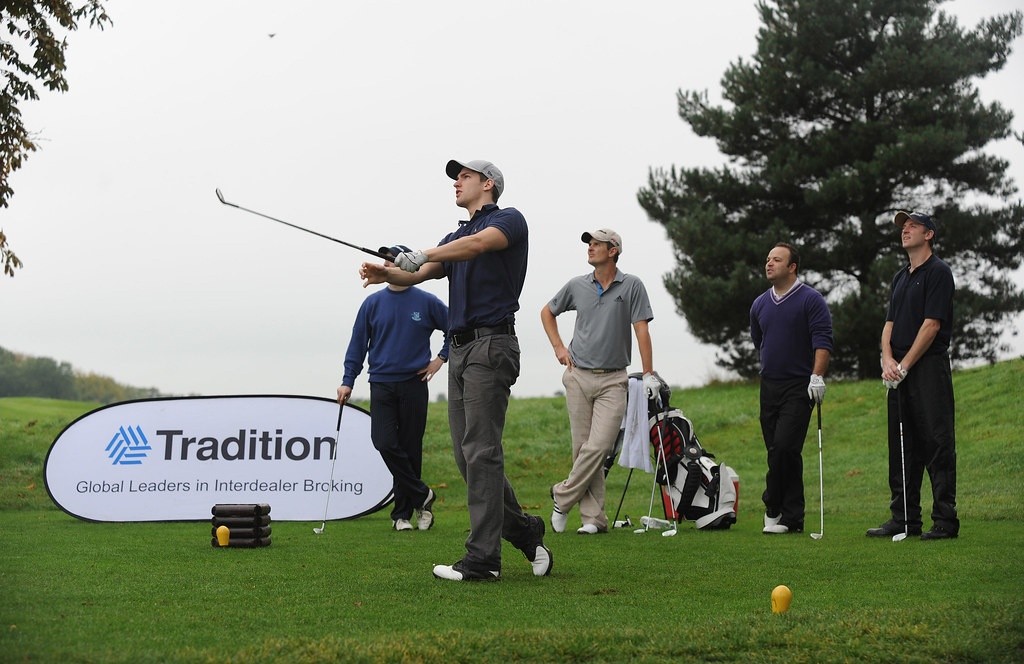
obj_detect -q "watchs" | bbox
[439,354,447,363]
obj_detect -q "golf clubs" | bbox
[891,385,909,541]
[313,396,348,534]
[811,397,825,540]
[632,388,678,537]
[215,186,421,273]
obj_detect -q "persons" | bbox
[359,160,553,583]
[865,211,960,541]
[750,243,833,534]
[541,228,661,534]
[336,245,450,532]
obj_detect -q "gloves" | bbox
[807,374,825,406]
[643,372,661,400]
[394,249,429,274]
[882,364,907,390]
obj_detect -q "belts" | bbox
[592,369,616,373]
[451,325,515,349]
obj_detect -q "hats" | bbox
[379,245,412,258]
[581,228,622,255]
[894,212,937,243]
[445,159,505,197]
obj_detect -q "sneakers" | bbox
[865,525,922,538]
[576,523,599,534]
[417,488,435,531]
[390,519,414,532]
[523,515,553,579]
[434,564,500,581]
[920,526,950,541]
[550,484,567,533]
[764,511,783,528]
[762,525,802,535]
